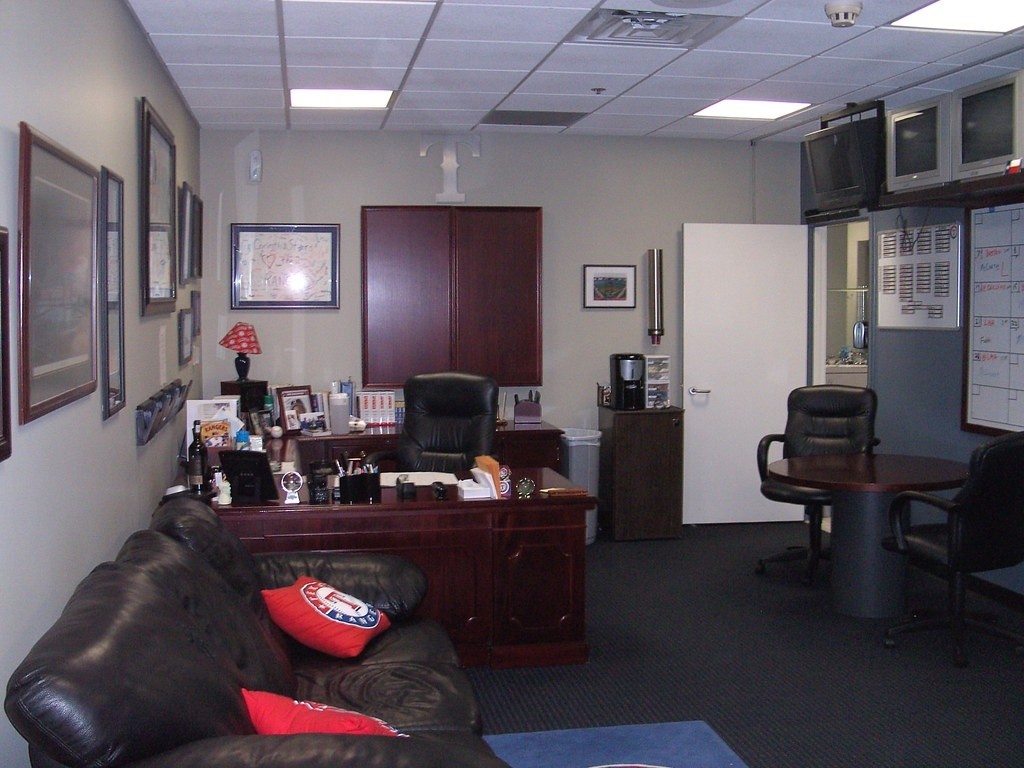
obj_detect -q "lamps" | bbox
[220,322,260,380]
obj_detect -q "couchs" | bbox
[4,498,506,768]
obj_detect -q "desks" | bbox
[167,467,598,668]
[598,406,684,541]
[221,380,269,413]
[207,419,565,468]
[769,454,967,621]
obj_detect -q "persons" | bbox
[289,398,307,421]
[287,413,299,430]
[301,413,327,431]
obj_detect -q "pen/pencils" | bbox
[512,390,542,404]
[333,455,380,476]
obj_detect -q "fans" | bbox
[281,472,303,504]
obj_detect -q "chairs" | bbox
[881,429,1024,652]
[363,372,507,475]
[754,385,881,586]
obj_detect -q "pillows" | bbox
[261,575,390,658]
[240,687,408,736]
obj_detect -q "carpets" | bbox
[483,719,746,768]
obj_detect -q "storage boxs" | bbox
[645,381,671,409]
[643,354,671,382]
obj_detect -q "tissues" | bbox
[456,478,491,499]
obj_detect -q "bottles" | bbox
[188,420,209,501]
[235,430,251,451]
[264,395,274,416]
[329,392,350,435]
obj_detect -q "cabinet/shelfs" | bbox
[360,205,542,387]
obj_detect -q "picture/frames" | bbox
[232,222,341,310]
[178,308,195,366]
[179,178,193,285]
[191,289,204,335]
[583,263,638,310]
[277,385,315,435]
[193,193,204,278]
[15,121,98,425]
[0,225,11,460]
[141,96,177,316]
[97,163,126,423]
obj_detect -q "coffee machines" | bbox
[609,352,646,411]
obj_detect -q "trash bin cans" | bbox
[557,427,602,546]
